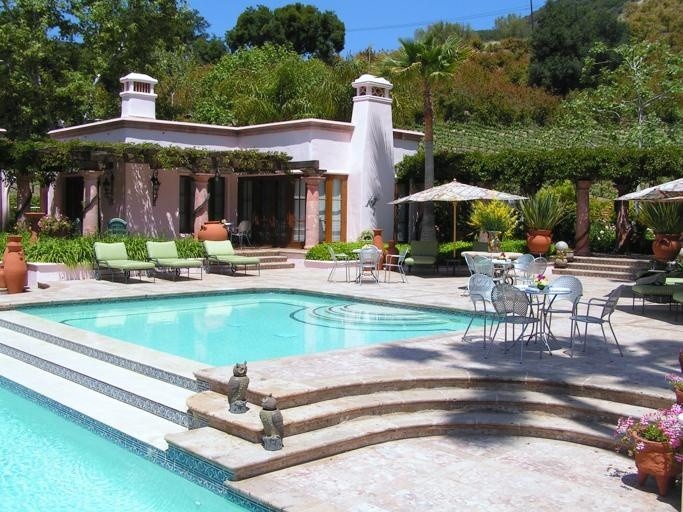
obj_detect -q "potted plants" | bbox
[674,388,683,407]
[626,197,683,262]
[678,352,682,372]
[513,187,573,256]
[464,195,516,255]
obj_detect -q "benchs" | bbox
[630,283,681,317]
[673,289,682,323]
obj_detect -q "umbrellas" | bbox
[611,177,682,207]
[380,178,530,258]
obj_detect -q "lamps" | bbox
[150,168,162,206]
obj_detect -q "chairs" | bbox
[455,249,625,365]
[202,239,261,278]
[145,241,202,281]
[229,221,251,249]
[109,218,127,233]
[326,242,409,284]
[405,239,440,275]
[93,241,155,284]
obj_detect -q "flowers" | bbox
[612,403,683,466]
[665,372,683,392]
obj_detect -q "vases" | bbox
[630,430,681,496]
[0,233,29,295]
[197,219,229,244]
